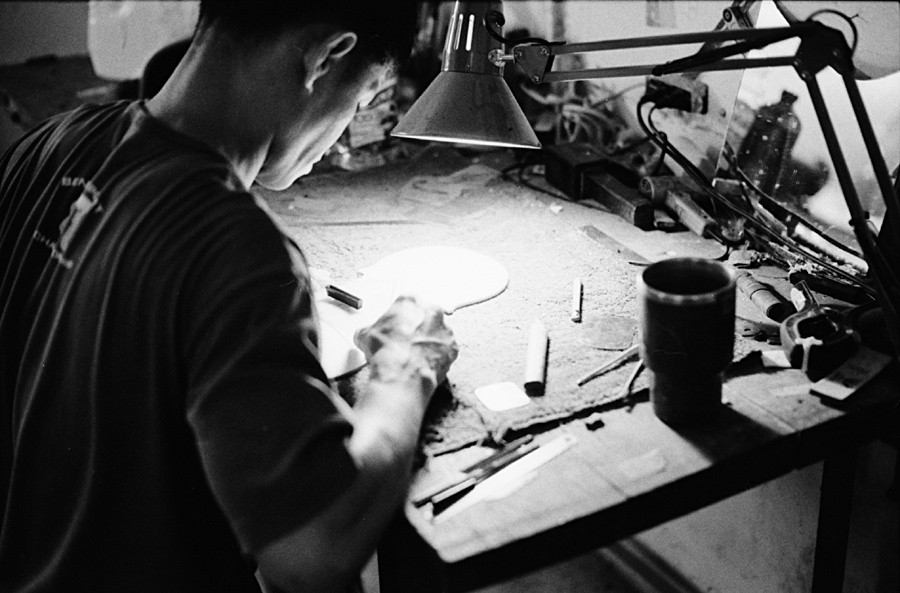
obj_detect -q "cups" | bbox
[640,257,737,426]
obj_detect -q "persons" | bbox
[0,0,457,593]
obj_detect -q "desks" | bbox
[242,163,900,593]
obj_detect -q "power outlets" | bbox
[644,71,709,117]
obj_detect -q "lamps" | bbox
[386,2,899,353]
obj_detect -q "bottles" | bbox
[737,89,803,201]
